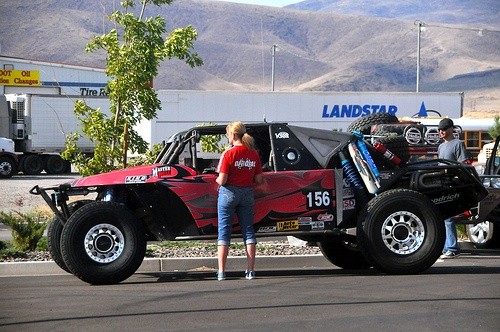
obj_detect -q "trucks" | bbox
[0,89,115,180]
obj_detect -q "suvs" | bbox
[345,113,474,167]
[26,119,490,287]
[448,133,500,249]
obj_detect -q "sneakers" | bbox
[244,269,255,279]
[439,249,461,259]
[216,271,227,280]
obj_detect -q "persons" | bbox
[215,121,262,280]
[437,119,470,258]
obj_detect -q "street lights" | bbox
[269,43,280,91]
[413,19,427,93]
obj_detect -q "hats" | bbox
[437,118,453,131]
